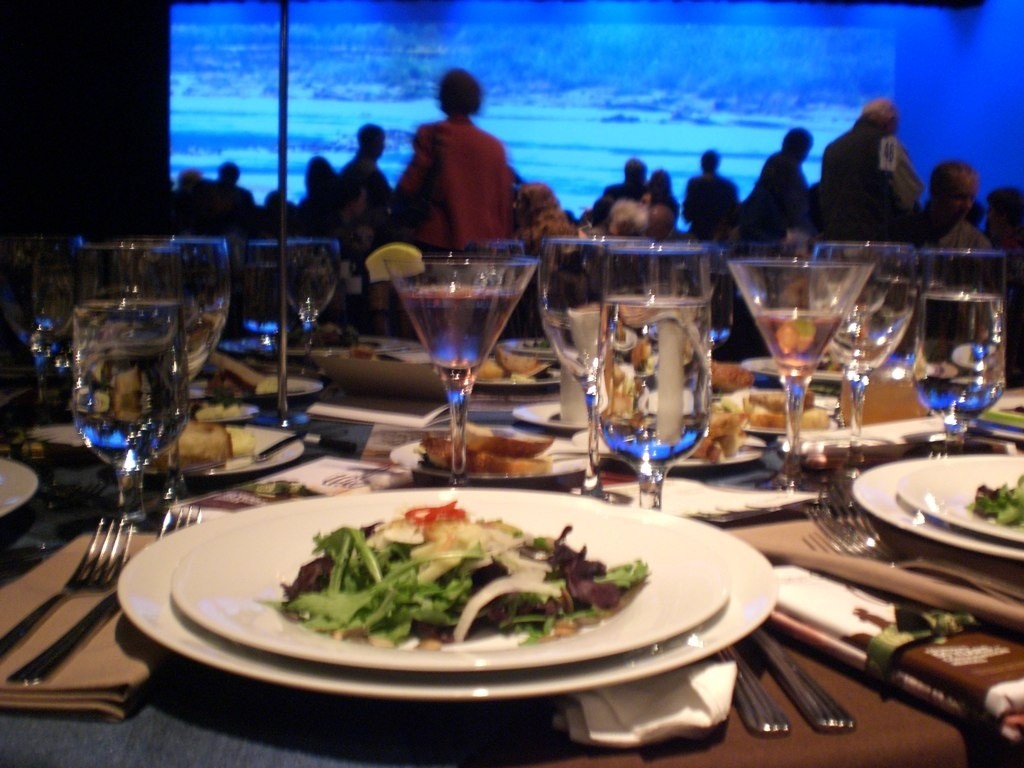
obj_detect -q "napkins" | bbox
[0,530,165,719]
[730,517,1024,631]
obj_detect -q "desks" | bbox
[0,340,1024,768]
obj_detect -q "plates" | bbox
[294,334,429,366]
[119,488,778,700]
[472,375,558,385]
[851,453,1024,558]
[201,424,304,475]
[0,458,39,513]
[389,436,588,480]
[574,428,768,467]
[967,384,1024,440]
[207,349,324,397]
[509,402,588,428]
[732,386,840,434]
[744,357,936,381]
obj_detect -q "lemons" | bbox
[366,244,426,281]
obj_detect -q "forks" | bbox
[806,481,1024,605]
[927,452,948,459]
[0,504,205,682]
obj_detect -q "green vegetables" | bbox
[965,470,1024,532]
[269,520,651,641]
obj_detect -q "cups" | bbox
[82,242,187,353]
[658,239,735,349]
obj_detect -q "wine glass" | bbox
[728,244,1007,493]
[588,245,715,510]
[538,238,654,504]
[385,255,544,489]
[244,240,336,379]
[0,236,229,504]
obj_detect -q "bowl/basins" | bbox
[497,341,553,375]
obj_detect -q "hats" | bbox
[863,98,899,119]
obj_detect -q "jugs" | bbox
[543,303,688,444]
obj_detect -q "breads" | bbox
[149,421,234,470]
[494,346,535,374]
[744,388,830,429]
[420,422,556,478]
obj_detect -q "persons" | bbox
[178,123,393,235]
[885,161,1024,255]
[392,67,514,247]
[514,182,580,306]
[565,158,680,328]
[681,127,814,241]
[811,99,923,242]
